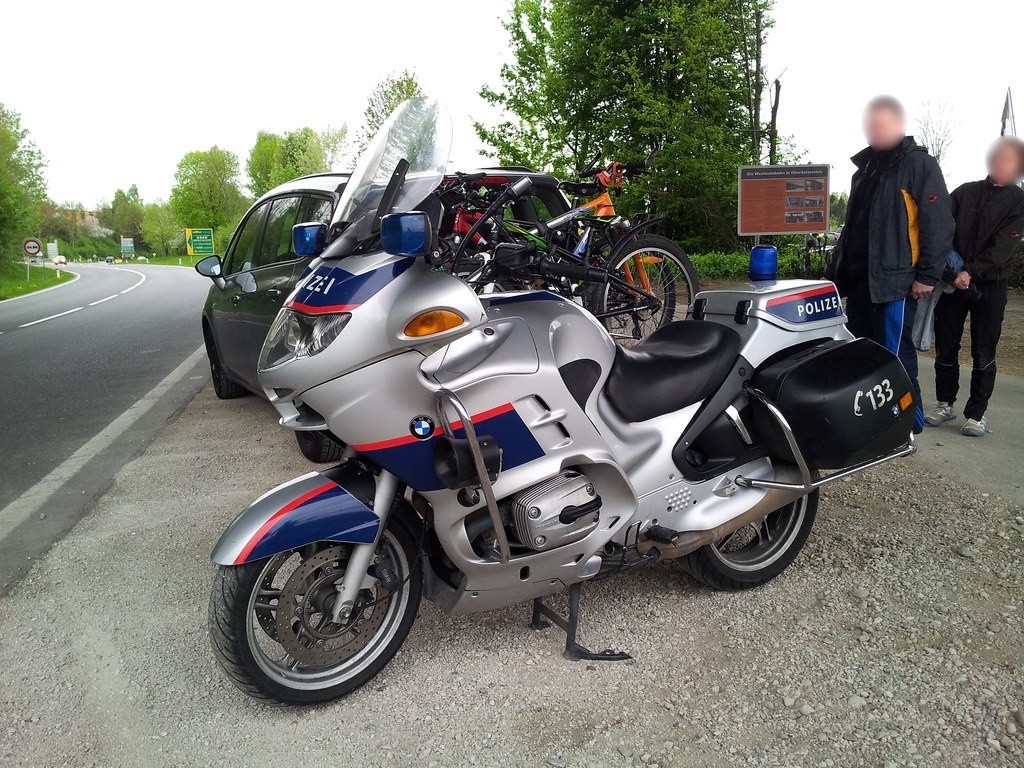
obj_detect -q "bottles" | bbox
[572,226,597,261]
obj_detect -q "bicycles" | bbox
[430,132,701,350]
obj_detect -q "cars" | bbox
[106,255,114,263]
[53,254,67,265]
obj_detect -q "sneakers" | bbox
[960,411,987,436]
[924,402,956,426]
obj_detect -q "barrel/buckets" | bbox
[748,244,779,282]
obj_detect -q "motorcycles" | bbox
[201,92,919,707]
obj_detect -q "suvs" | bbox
[193,164,612,467]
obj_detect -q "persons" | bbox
[836,96,956,434]
[924,136,1023,432]
